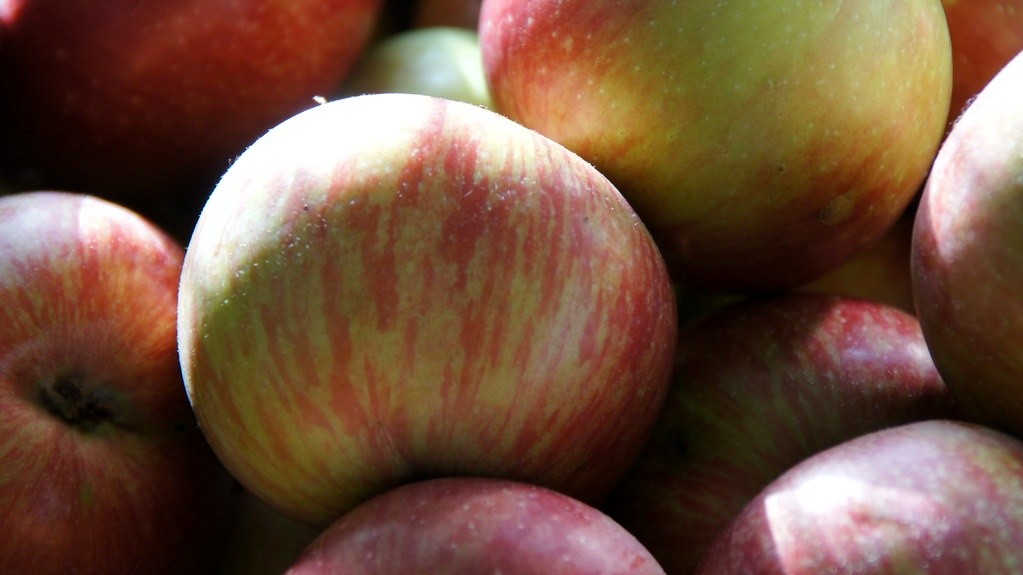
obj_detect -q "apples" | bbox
[0,0,1023,575]
